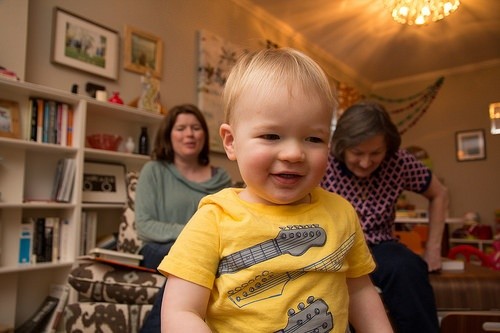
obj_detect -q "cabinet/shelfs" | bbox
[1,72,166,332]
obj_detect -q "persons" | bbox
[135,103,235,333]
[318,102,448,333]
[157,47,394,333]
[137,70,161,115]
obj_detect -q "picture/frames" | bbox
[454,128,486,161]
[49,5,121,81]
[81,158,130,204]
[120,22,164,80]
[488,102,500,134]
[195,27,249,154]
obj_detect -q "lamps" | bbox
[390,0,461,26]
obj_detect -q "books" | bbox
[0,95,118,266]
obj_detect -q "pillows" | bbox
[66,259,164,305]
